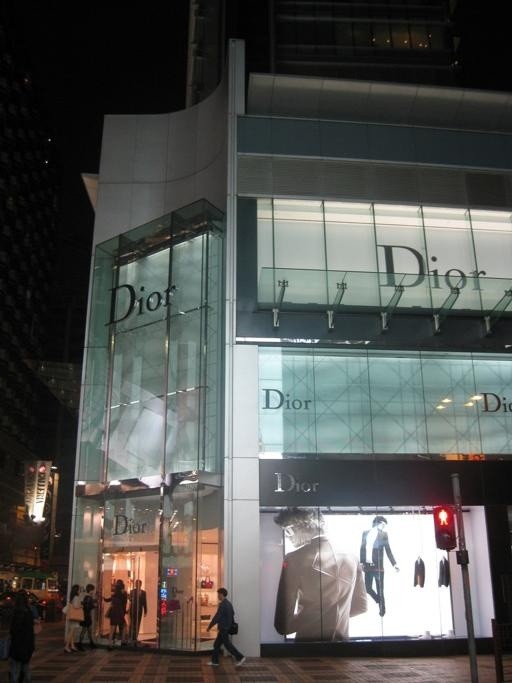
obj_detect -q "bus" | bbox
[9,572,58,603]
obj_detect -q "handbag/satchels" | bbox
[67,605,84,622]
[0,639,11,659]
[229,622,238,635]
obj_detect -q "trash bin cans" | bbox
[47,598,63,622]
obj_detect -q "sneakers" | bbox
[236,656,246,666]
[208,661,219,666]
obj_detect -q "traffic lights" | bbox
[433,506,457,551]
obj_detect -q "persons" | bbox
[206,587,246,667]
[360,515,401,617]
[6,590,35,683]
[273,509,367,642]
[54,573,148,653]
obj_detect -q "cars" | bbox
[1,590,45,620]
